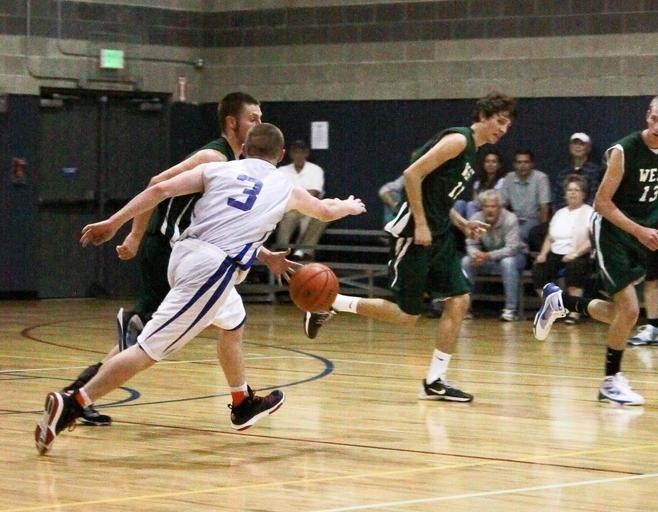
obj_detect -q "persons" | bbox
[532,95,658,407]
[30,121,369,455]
[377,177,410,226]
[265,137,327,304]
[452,151,552,321]
[305,90,519,403]
[63,90,305,432]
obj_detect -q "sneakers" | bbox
[34,390,84,457]
[60,383,113,428]
[627,323,658,348]
[597,370,647,408]
[564,311,583,325]
[116,305,147,352]
[304,305,337,339]
[228,386,286,432]
[532,281,571,342]
[500,308,519,320]
[416,378,473,403]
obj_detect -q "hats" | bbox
[570,132,591,144]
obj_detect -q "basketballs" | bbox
[290,264,338,312]
